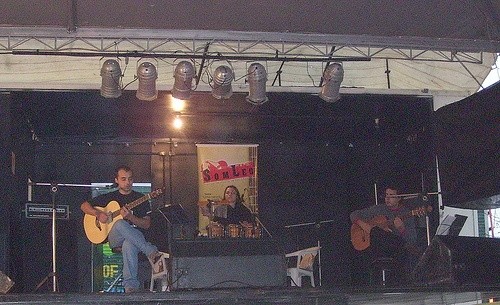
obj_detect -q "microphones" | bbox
[241,188,245,197]
[110,183,119,188]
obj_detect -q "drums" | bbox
[207,223,228,238]
[239,224,264,239]
[225,224,242,239]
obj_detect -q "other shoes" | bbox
[149,251,163,264]
[126,287,138,296]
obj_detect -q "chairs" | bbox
[150,252,169,293]
[286,246,321,287]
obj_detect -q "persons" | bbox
[80,166,164,293]
[200,186,257,227]
[350,185,417,265]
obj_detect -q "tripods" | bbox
[31,183,112,294]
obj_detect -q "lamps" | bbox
[136,57,158,101]
[210,59,235,100]
[318,63,345,103]
[99,56,122,98]
[171,58,198,101]
[244,59,270,106]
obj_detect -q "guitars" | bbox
[350,205,435,251]
[82,186,169,245]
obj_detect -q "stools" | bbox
[106,248,141,292]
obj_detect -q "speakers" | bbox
[0,270,16,293]
[407,235,500,287]
[167,237,288,291]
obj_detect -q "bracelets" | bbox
[95,209,99,215]
